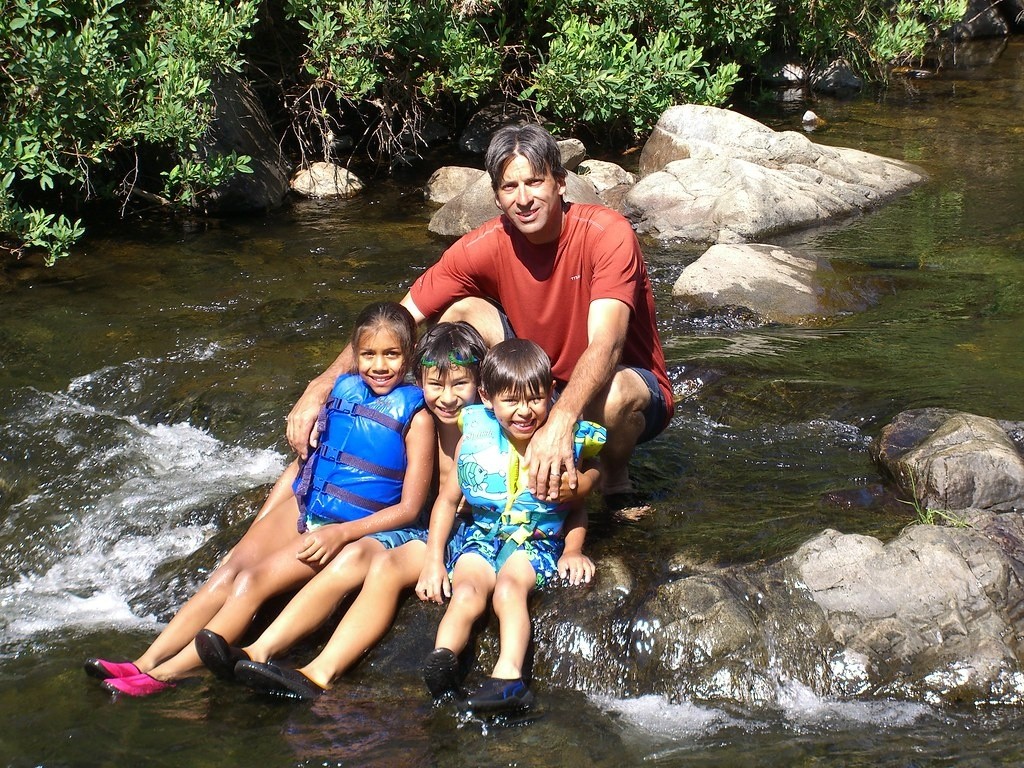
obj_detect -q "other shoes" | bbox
[458,678,533,711]
[84,659,142,680]
[102,673,177,699]
[236,660,324,701]
[197,629,253,685]
[423,648,461,699]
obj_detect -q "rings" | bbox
[550,473,561,476]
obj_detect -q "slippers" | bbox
[604,491,643,523]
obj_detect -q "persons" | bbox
[83,302,436,699]
[414,339,607,714]
[286,126,673,500]
[194,319,491,699]
